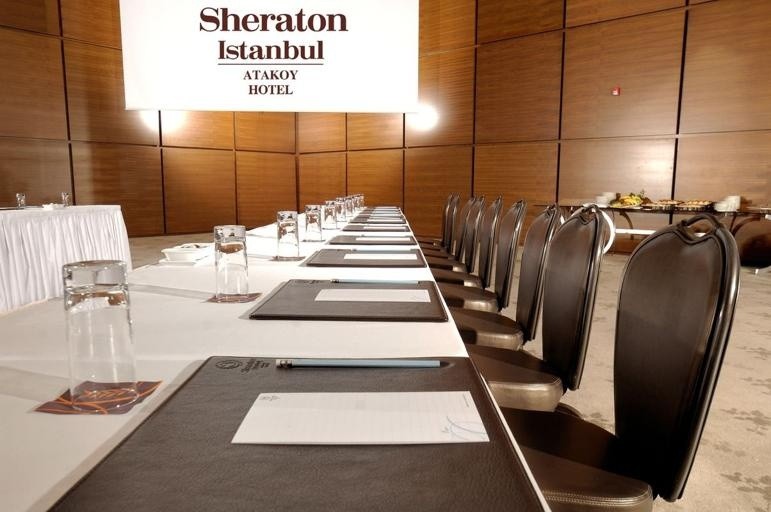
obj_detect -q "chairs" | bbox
[1,191,771,512]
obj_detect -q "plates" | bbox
[41,203,65,210]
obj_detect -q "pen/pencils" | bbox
[362,234,409,237]
[349,248,413,251]
[276,359,453,368]
[331,278,421,284]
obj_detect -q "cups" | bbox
[304,204,322,243]
[61,260,140,413]
[15,192,26,210]
[213,225,250,304]
[345,197,352,218]
[62,192,71,207]
[324,201,337,231]
[276,210,299,263]
[336,197,347,222]
[352,193,365,214]
